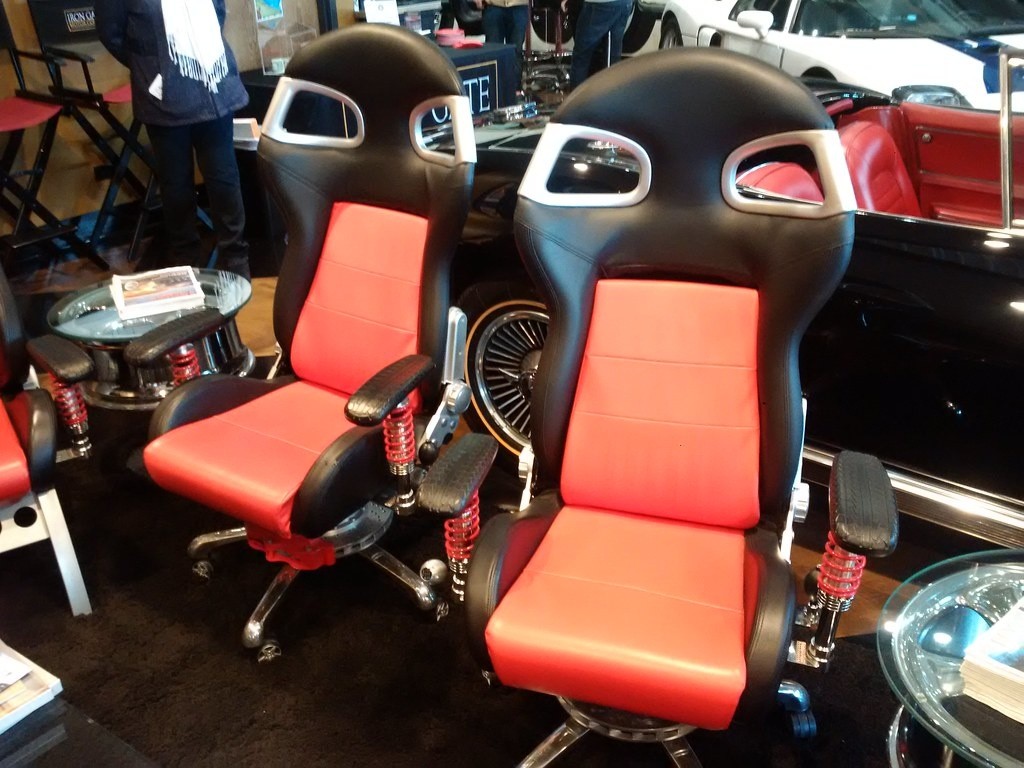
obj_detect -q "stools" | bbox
[43,46,215,262]
[0,47,112,272]
[520,0,575,89]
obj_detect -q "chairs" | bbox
[838,119,922,218]
[736,161,825,202]
[0,266,95,620]
[416,47,897,768]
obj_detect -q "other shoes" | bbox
[158,255,188,269]
[223,256,250,276]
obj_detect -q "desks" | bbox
[144,24,477,654]
[46,268,257,410]
[876,549,1024,768]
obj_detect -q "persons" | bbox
[94,0,251,282]
[474,0,530,101]
[560,0,638,86]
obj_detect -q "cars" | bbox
[656,0,1024,123]
